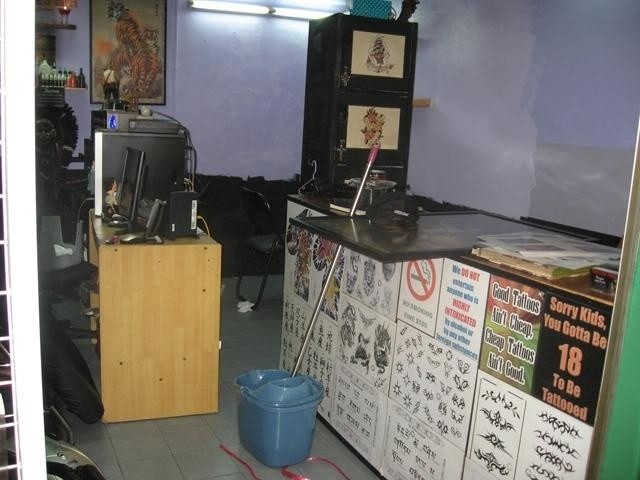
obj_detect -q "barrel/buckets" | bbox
[234,367,326,467]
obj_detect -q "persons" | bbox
[102,65,121,110]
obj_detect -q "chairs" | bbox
[193,172,300,309]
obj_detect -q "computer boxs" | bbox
[94,130,187,217]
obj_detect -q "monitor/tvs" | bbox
[117,147,146,234]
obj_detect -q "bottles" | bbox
[38,52,85,88]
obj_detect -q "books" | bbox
[470,243,619,280]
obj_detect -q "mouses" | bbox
[120,233,146,244]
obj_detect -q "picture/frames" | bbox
[88,0,167,105]
[290,209,603,262]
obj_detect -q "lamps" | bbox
[187,0,334,21]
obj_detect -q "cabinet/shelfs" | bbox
[278,191,624,480]
[89,209,223,424]
[298,14,418,198]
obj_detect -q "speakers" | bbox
[163,190,198,240]
